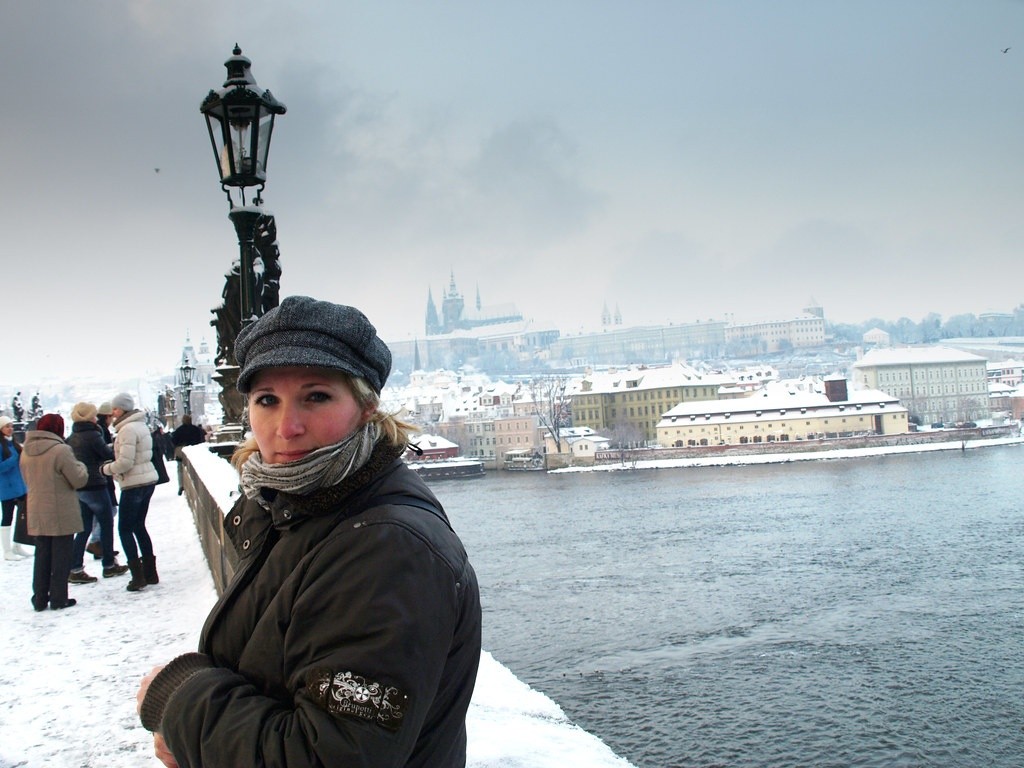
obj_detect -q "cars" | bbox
[930,421,978,429]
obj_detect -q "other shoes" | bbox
[178,487,184,495]
[94,542,119,560]
[34,604,48,612]
[86,544,93,553]
[55,599,76,609]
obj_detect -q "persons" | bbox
[87,403,120,560]
[146,410,213,496]
[65,402,128,584]
[137,296,482,768]
[19,413,89,611]
[99,393,159,591]
[0,416,28,562]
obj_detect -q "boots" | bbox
[0,525,22,561]
[129,556,159,584]
[13,543,32,557]
[127,559,147,591]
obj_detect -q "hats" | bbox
[112,393,134,411]
[0,416,11,429]
[36,413,64,439]
[96,401,113,415]
[233,296,393,398]
[71,402,97,422]
[181,414,192,424]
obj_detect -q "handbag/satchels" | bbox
[13,502,35,545]
[150,430,170,485]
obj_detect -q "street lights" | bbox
[177,355,197,416]
[198,42,288,327]
[168,396,178,430]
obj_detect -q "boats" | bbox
[507,456,544,471]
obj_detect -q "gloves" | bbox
[98,460,112,481]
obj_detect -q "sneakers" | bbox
[103,564,128,578]
[68,571,97,584]
